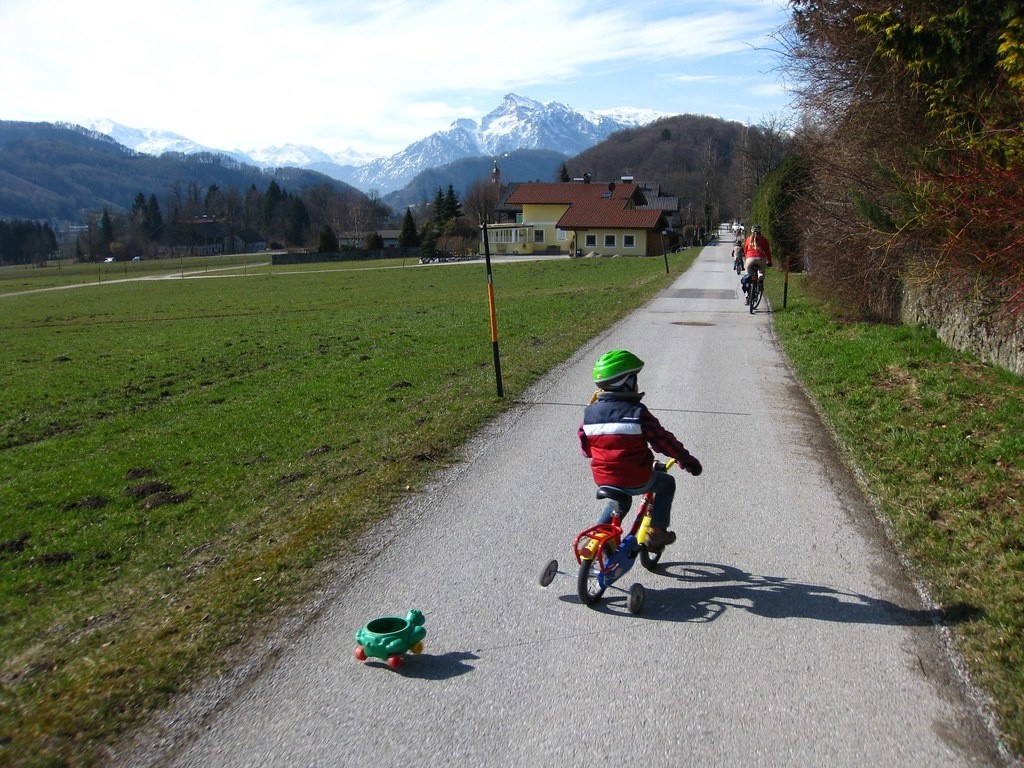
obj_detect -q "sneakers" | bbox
[646,528,676,544]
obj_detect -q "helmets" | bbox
[593,350,645,392]
[751,224,762,232]
[736,241,742,245]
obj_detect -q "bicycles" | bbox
[746,265,767,313]
[736,260,741,275]
[538,456,680,613]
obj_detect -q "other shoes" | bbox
[744,300,749,305]
[757,279,764,291]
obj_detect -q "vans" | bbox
[104,257,116,263]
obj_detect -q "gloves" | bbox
[678,455,702,476]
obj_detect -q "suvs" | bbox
[132,256,144,262]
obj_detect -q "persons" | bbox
[577,350,702,550]
[735,227,743,246]
[742,224,772,305]
[732,240,745,271]
